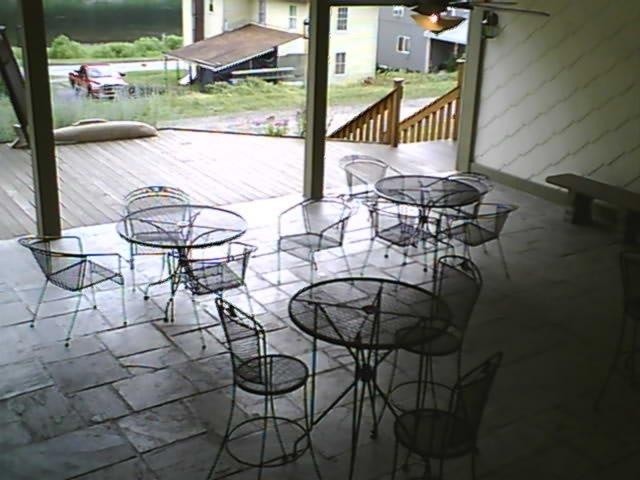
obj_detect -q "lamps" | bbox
[409,1,469,40]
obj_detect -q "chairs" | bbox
[392,350,500,480]
[206,299,321,480]
[339,154,519,278]
[275,194,353,292]
[165,241,257,347]
[592,249,640,426]
[121,185,199,290]
[373,254,483,435]
[18,235,128,347]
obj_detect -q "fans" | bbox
[332,0,550,37]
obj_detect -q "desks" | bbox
[115,204,249,322]
[284,276,452,478]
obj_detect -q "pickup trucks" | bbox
[68,63,128,98]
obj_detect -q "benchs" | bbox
[545,174,639,227]
